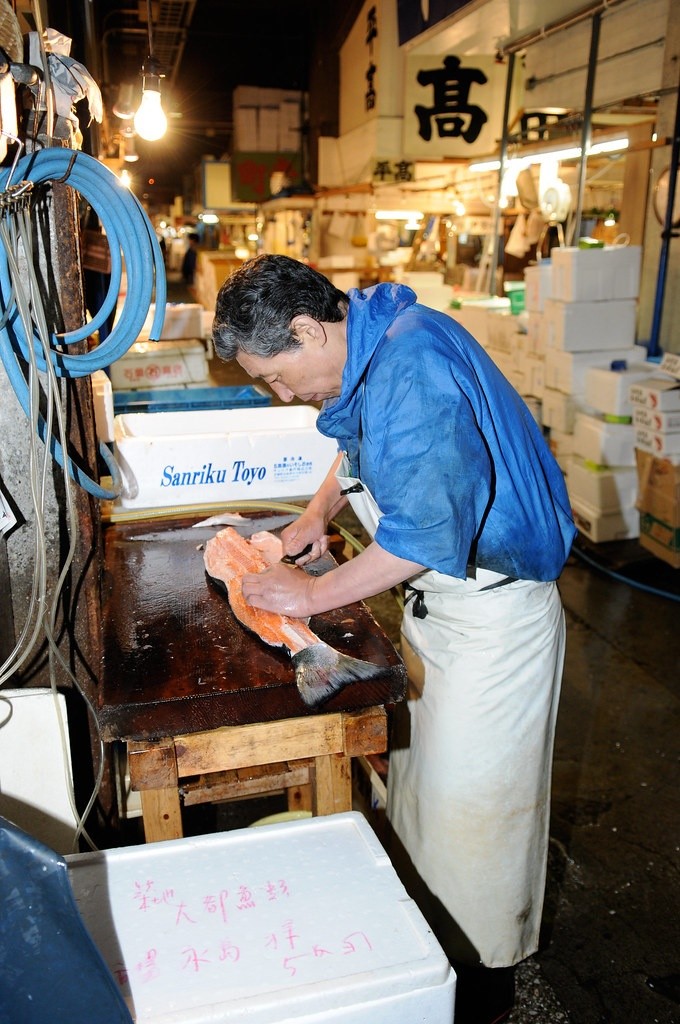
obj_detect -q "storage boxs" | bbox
[394,244,680,568]
[0,688,457,1024]
[87,246,342,508]
[325,254,359,295]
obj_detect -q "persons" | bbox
[181,232,202,290]
[216,253,576,1024]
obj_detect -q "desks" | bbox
[96,519,410,845]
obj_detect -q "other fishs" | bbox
[202,523,395,707]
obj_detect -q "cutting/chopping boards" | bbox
[93,510,413,740]
